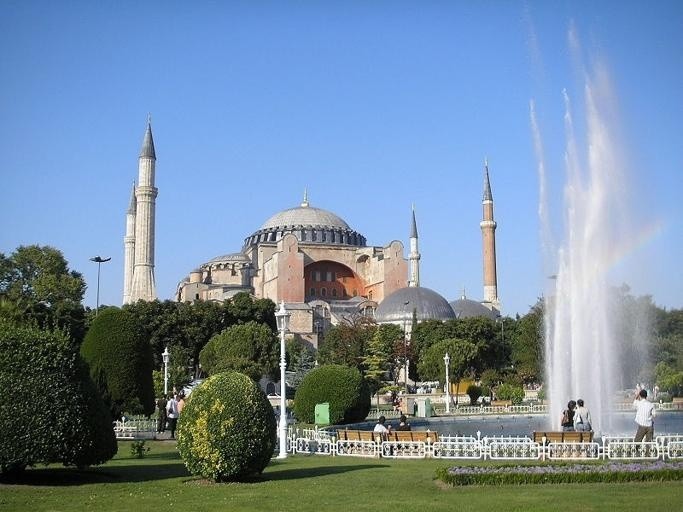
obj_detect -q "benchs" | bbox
[335,426,387,446]
[387,428,440,449]
[532,428,595,446]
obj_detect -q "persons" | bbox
[406,382,439,394]
[392,398,398,413]
[156,389,187,438]
[572,399,592,431]
[560,400,577,432]
[395,415,410,431]
[630,388,657,456]
[372,415,391,443]
[397,397,402,413]
[272,404,299,422]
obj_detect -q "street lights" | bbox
[161,347,172,398]
[89,255,111,316]
[271,299,293,460]
[441,350,450,415]
[402,298,410,396]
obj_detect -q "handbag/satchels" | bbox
[560,410,570,426]
[576,415,581,424]
